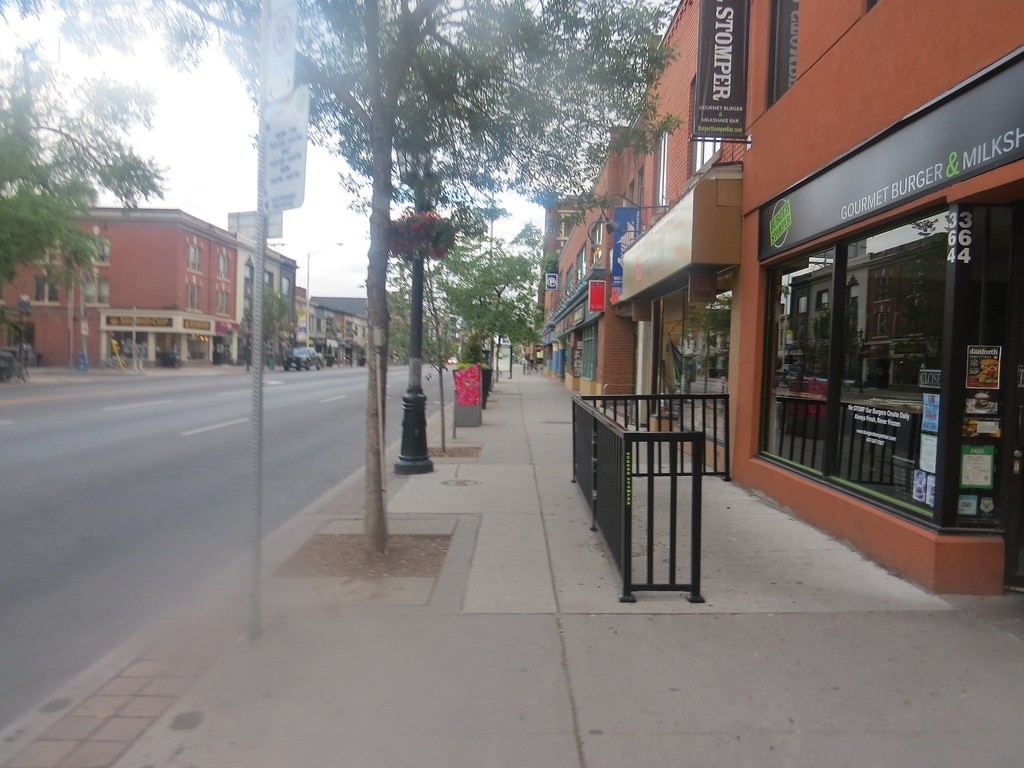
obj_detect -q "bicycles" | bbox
[3,360,30,383]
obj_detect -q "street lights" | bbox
[392,136,434,475]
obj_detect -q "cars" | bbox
[282,346,321,370]
